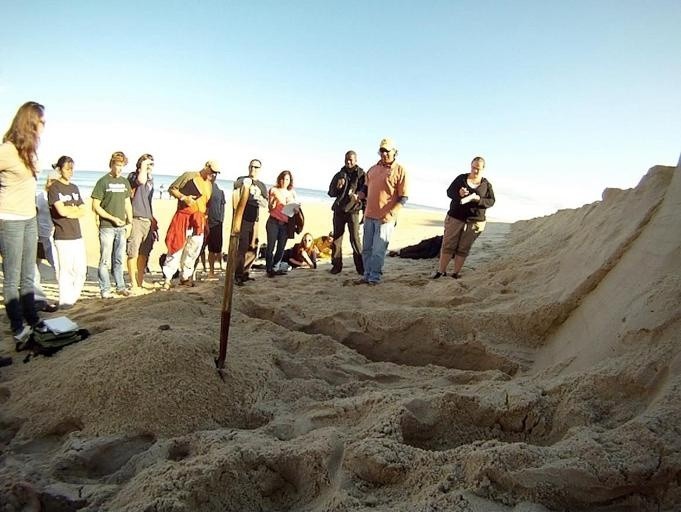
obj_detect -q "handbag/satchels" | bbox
[17,322,88,358]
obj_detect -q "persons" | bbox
[161,159,336,291]
[1,100,160,340]
[329,139,411,286]
[433,156,496,279]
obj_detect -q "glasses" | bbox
[304,238,311,241]
[251,166,259,168]
[40,120,45,126]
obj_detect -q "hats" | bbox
[379,139,394,151]
[206,161,220,173]
